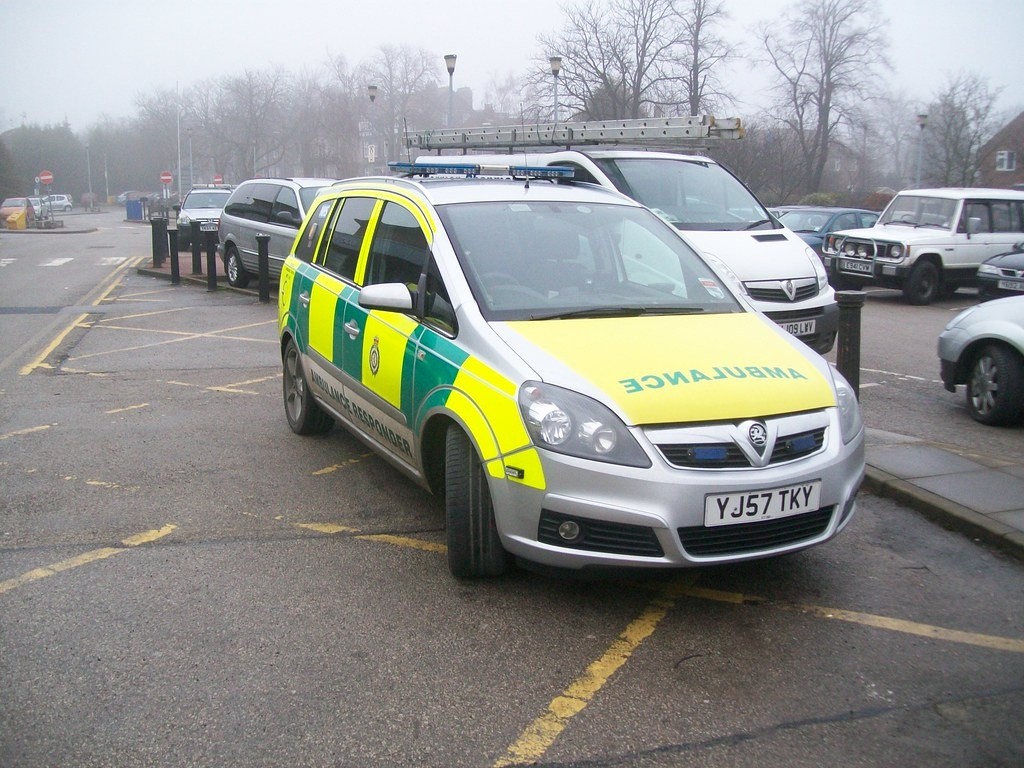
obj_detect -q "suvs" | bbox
[821,186,1024,306]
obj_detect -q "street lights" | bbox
[367,84,378,145]
[915,113,929,186]
[444,54,458,127]
[549,56,563,120]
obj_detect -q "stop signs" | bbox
[214,175,223,184]
[39,170,54,185]
[159,171,173,184]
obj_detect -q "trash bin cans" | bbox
[127,199,143,221]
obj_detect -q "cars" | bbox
[117,189,178,207]
[26,197,50,221]
[729,204,881,261]
[0,198,36,228]
[277,160,867,581]
[976,241,1024,302]
[937,295,1024,426]
[216,178,344,289]
[42,194,73,213]
[177,183,238,253]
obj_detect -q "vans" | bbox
[412,149,842,358]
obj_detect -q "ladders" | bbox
[400,114,743,151]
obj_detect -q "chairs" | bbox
[805,216,823,230]
